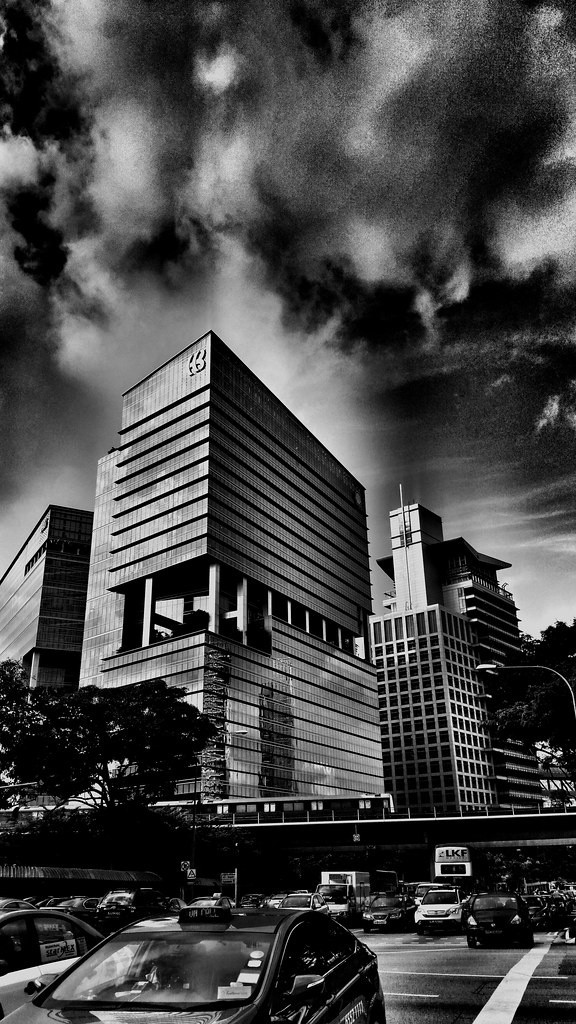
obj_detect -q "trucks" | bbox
[315,871,371,926]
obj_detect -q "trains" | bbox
[0,792,395,830]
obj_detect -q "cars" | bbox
[363,880,534,949]
[0,887,384,1024]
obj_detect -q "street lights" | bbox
[475,663,576,721]
